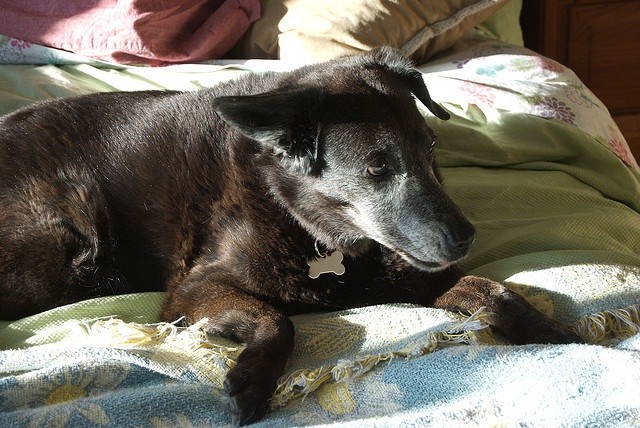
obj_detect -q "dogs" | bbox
[0,45,585,428]
